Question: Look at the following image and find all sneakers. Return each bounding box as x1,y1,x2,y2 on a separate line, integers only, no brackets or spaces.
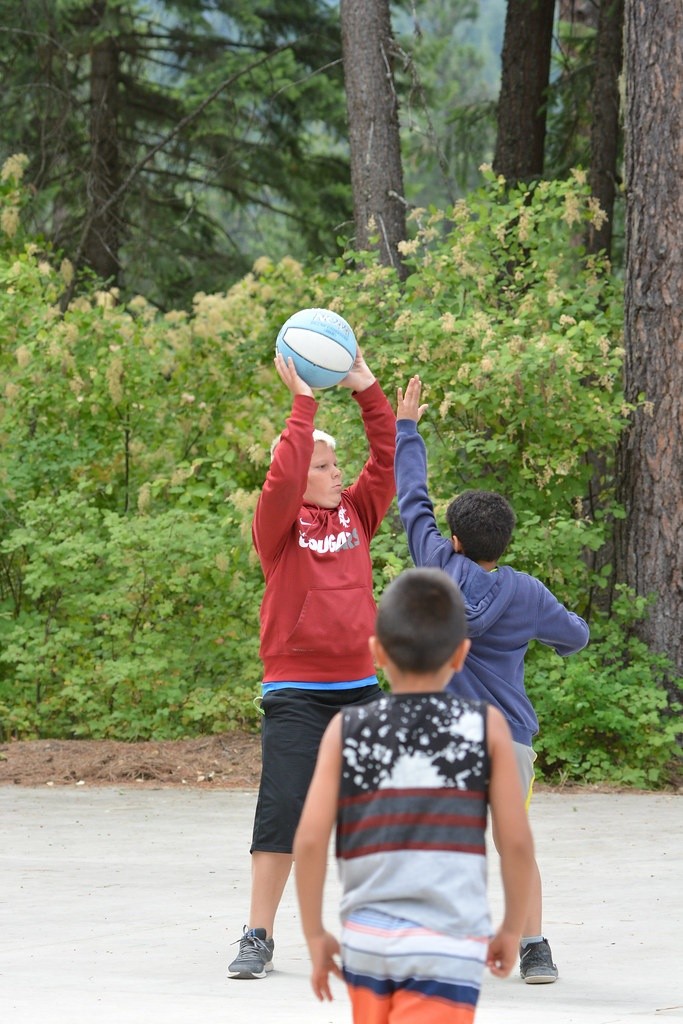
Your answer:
520,937,558,983
227,925,275,978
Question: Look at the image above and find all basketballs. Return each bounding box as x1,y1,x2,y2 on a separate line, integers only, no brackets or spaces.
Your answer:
274,306,359,393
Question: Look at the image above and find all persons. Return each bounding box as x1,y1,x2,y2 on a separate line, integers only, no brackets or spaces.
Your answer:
394,374,590,984
293,566,534,1024
226,343,397,980
